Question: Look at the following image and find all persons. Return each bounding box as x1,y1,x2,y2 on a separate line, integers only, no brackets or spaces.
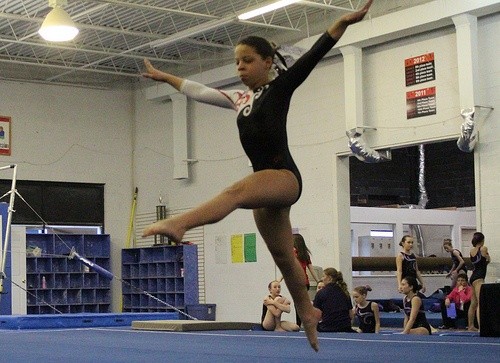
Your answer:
352,285,380,333
467,232,490,331
443,239,467,287
400,276,431,335
310,280,326,305
444,274,473,329
279,234,320,327
261,280,300,333
396,235,426,294
142,0,372,352
313,268,353,333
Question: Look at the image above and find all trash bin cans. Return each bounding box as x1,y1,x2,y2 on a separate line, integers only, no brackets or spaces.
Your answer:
185,303,216,320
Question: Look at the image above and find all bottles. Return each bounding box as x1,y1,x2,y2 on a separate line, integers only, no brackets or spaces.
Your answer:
180,266,184,277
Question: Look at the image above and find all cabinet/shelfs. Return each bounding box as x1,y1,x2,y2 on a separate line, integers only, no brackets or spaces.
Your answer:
122,245,198,312
26,233,114,312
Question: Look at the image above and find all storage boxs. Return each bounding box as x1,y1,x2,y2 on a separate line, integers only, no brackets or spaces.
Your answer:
185,304,216,321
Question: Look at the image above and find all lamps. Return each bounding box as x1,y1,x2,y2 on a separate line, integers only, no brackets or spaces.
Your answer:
38,0,80,42
148,13,237,49
235,0,299,21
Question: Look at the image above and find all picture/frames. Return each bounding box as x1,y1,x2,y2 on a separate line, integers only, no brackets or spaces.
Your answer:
0,116,12,156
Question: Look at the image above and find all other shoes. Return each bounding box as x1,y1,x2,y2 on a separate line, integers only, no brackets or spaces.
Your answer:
439,325,449,329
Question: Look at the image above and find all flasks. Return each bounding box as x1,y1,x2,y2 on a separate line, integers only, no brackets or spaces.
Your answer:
42,276,47,288
